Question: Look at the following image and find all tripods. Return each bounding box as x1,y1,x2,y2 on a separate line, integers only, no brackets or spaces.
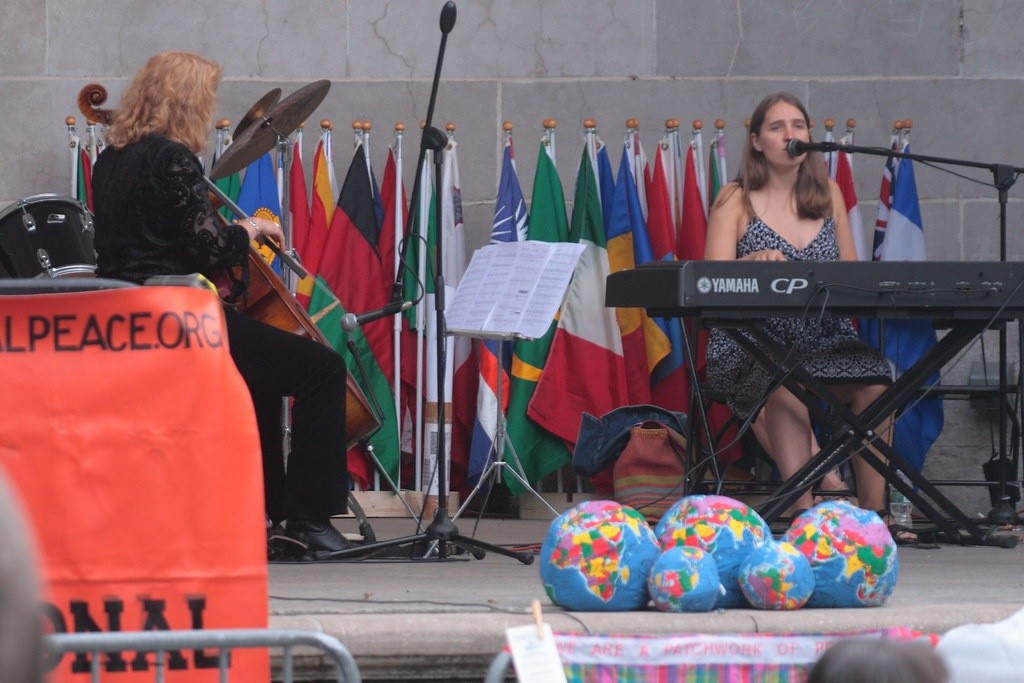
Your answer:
312,0,591,564
822,143,1024,543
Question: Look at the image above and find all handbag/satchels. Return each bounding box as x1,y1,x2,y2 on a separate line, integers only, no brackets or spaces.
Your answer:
614,420,715,522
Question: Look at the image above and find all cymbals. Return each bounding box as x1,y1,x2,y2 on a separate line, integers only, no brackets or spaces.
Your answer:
209,77,333,181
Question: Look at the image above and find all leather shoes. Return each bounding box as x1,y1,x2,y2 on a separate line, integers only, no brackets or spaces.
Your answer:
285,518,381,562
266,522,285,557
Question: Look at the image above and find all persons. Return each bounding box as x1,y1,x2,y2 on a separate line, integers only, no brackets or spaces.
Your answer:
808,637,949,683
704,92,921,547
90,53,369,559
0,465,44,683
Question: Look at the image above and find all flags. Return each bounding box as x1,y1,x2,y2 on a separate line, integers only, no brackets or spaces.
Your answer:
67,115,947,499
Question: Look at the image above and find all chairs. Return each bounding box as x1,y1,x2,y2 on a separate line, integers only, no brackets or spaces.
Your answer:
683,317,890,498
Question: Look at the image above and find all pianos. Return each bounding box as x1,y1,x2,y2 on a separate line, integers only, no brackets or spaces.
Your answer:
602,258,1024,321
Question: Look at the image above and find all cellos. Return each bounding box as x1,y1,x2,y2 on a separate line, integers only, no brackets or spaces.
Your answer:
76,82,441,555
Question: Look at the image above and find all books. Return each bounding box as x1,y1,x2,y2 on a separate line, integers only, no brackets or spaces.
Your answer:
446,240,585,337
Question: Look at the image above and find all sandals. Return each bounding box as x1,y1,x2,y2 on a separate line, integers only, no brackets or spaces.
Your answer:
877,509,919,544
789,509,808,528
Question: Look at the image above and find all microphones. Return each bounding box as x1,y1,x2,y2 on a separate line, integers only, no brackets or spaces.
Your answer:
340,299,420,333
786,138,838,156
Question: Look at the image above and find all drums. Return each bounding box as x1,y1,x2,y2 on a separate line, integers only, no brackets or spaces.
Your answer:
0,191,98,280
